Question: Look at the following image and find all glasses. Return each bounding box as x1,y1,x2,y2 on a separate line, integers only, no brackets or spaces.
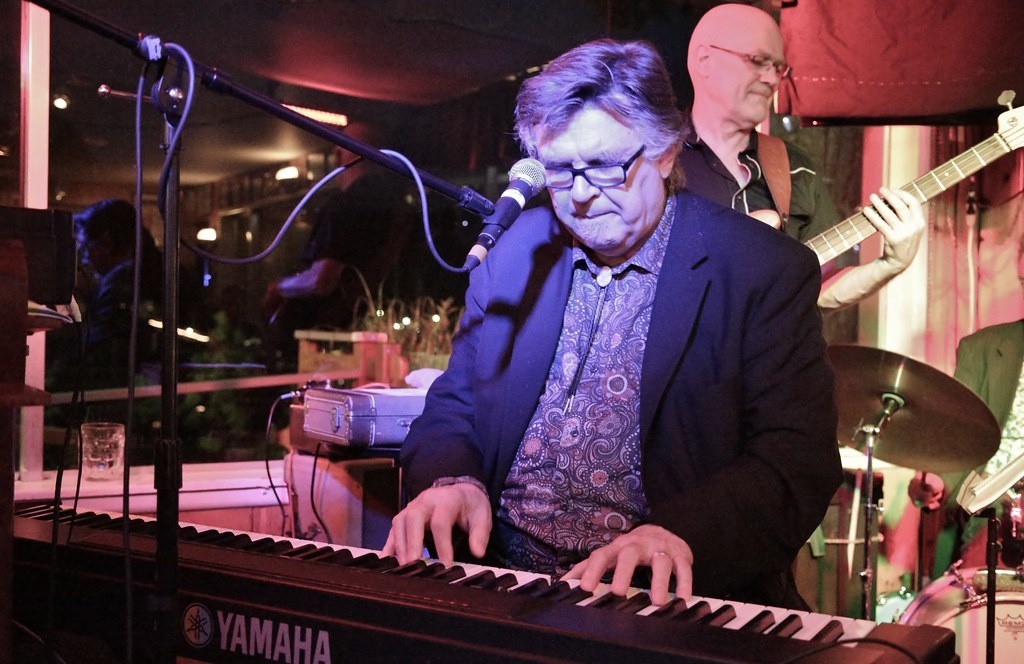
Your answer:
710,44,793,79
543,144,646,189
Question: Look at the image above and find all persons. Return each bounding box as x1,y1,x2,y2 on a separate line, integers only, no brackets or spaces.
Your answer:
265,123,395,372
73,199,164,393
909,233,1024,569
382,38,844,605
675,4,926,315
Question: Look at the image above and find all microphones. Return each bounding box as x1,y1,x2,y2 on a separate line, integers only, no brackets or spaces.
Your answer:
465,158,548,272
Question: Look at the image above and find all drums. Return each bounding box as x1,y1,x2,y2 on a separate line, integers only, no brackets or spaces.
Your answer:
897,564,1024,663
792,469,883,619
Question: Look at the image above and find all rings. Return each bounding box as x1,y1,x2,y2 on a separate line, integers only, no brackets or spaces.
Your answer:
651,549,667,567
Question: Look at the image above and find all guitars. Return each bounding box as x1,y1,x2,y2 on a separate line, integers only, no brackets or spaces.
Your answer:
802,87,1024,266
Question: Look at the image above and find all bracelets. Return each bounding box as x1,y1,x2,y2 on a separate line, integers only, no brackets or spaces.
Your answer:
431,474,490,498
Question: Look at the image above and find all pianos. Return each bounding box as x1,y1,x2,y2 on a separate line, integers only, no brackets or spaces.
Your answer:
10,499,957,663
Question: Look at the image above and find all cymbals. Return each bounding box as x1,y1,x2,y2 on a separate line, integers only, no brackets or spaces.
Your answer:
828,344,1001,471
